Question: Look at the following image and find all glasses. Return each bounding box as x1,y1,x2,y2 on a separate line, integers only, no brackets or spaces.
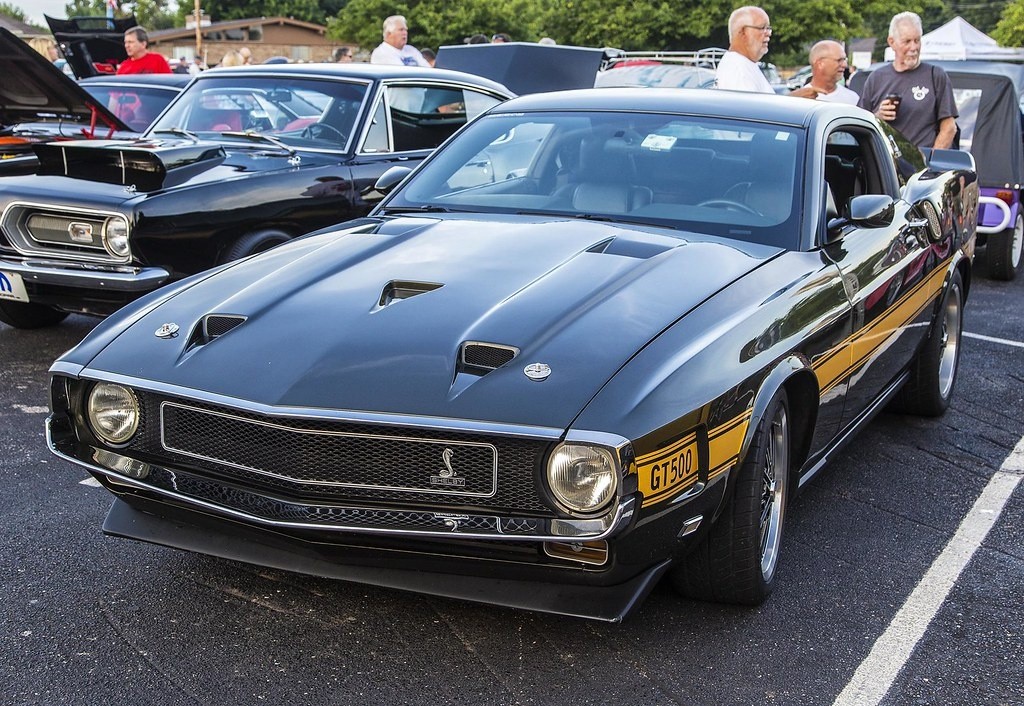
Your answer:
739,24,773,34
820,55,849,65
344,53,353,59
492,35,507,42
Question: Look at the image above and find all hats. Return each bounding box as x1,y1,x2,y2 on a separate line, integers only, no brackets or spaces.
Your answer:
463,34,490,44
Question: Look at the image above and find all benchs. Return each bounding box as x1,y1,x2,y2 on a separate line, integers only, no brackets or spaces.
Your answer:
632,145,854,222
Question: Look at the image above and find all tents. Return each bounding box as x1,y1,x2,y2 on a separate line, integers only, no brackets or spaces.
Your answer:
884,16,997,62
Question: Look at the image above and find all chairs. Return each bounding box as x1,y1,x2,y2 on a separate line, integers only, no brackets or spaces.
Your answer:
719,130,797,222
551,135,653,215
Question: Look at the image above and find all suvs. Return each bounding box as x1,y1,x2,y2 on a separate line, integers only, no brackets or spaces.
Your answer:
592,46,789,102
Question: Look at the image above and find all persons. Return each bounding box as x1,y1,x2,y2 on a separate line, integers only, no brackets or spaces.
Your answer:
860,12,959,150
846,66,858,86
803,40,860,145
54,25,251,129
714,6,818,141
335,15,556,115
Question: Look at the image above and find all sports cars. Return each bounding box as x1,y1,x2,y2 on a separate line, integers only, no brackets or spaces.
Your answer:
0,13,608,329
44,86,981,623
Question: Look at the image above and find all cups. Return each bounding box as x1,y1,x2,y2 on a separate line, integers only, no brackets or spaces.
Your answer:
886,95,902,122
